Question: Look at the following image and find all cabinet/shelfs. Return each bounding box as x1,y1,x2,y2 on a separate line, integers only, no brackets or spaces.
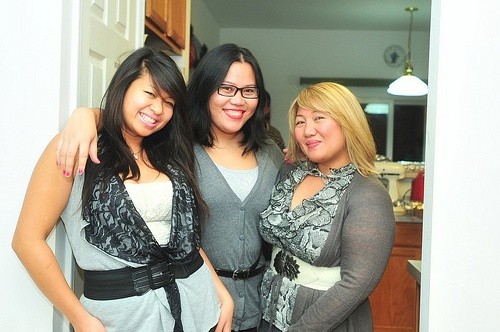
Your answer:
367,223,422,332
145,0,192,83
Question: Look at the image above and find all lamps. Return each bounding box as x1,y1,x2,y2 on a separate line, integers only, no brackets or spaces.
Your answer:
387,6,429,97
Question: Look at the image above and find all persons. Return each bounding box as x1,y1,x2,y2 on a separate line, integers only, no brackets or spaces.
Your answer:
55,43,308,332
259,81,396,332
11,47,235,332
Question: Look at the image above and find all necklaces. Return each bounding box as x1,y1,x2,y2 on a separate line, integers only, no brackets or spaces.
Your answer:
130,149,141,161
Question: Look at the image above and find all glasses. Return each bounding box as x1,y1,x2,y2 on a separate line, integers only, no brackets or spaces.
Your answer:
218,84,259,99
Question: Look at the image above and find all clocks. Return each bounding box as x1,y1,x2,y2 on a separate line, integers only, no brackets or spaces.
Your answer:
383,45,407,68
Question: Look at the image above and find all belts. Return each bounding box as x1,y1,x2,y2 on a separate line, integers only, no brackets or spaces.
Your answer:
214,264,265,280
